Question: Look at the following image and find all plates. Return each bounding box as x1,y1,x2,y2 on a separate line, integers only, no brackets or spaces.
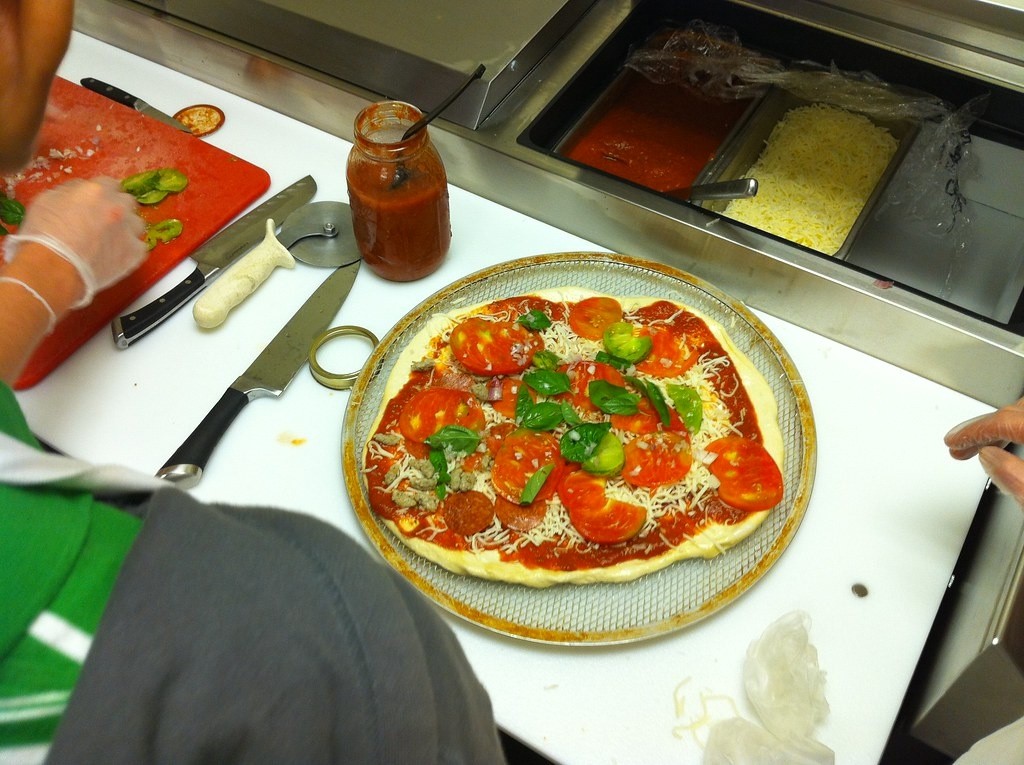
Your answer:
341,252,816,648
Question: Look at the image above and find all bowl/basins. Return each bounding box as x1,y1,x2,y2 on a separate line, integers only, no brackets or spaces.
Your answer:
695,62,917,259
550,28,773,202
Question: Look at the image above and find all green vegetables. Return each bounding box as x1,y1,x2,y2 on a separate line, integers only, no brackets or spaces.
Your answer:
0,191,26,236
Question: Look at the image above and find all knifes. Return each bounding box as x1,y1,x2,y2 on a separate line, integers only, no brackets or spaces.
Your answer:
154,260,360,489
112,176,317,348
80,77,194,134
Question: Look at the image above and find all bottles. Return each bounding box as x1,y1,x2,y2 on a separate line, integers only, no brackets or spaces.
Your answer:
347,101,451,282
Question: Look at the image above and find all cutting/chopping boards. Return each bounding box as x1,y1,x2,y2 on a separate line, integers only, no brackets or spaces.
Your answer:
0,75,268,390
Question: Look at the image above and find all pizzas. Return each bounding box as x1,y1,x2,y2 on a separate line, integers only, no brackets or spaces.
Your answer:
360,284,784,589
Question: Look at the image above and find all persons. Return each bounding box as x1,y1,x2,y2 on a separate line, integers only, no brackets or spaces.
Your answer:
945,395,1024,513
0,1,508,765
